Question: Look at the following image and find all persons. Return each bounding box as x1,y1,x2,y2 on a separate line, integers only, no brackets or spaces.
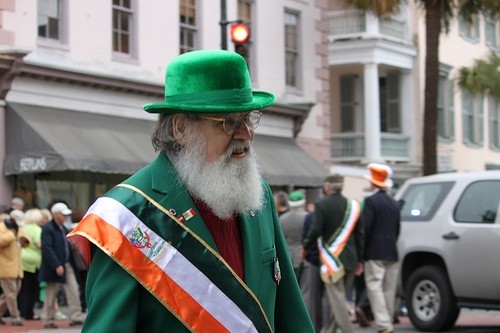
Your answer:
0,209,31,326
300,173,364,332
0,198,89,319
277,191,309,333
273,190,289,216
40,203,87,328
305,199,316,214
359,163,402,333
18,209,43,320
354,275,374,328
82,50,315,333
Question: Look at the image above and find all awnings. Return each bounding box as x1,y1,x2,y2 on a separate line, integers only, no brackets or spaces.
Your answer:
4,101,335,195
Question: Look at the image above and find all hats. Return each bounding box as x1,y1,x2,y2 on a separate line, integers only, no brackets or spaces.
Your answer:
51,202,72,215
363,164,393,188
324,173,345,182
287,191,306,206
142,49,275,112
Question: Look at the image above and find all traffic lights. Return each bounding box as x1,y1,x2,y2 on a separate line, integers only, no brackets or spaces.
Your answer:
230,23,249,42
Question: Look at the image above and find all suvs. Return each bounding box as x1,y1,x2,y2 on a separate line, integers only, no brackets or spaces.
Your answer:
393,170,500,332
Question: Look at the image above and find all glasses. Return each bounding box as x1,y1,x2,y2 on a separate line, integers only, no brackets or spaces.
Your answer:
197,110,264,134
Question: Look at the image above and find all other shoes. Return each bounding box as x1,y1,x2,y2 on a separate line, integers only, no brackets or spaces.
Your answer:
69,320,83,326
0,316,7,325
11,320,23,326
378,326,394,333
54,311,65,318
44,323,58,328
356,307,371,327
